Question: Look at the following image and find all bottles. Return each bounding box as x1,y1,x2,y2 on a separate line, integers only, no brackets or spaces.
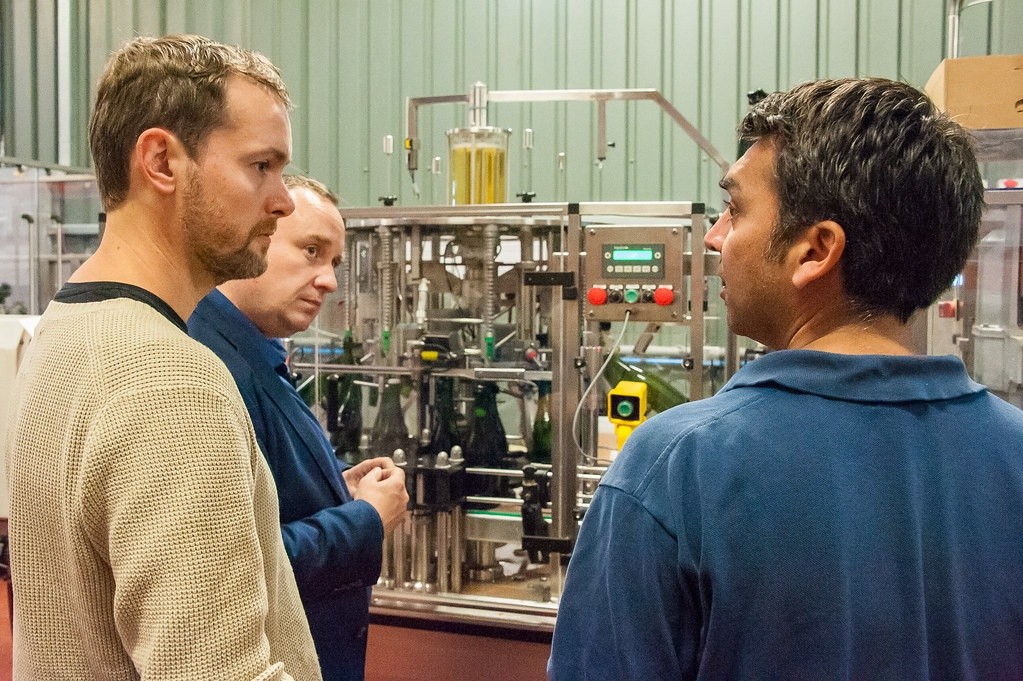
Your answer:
530,382,552,464
421,377,462,505
370,377,409,462
604,356,691,412
328,373,362,466
463,382,507,510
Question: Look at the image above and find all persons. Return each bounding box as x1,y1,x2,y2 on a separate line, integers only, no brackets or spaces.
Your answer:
8,28,329,681
546,73,1023,681
188,172,410,681
